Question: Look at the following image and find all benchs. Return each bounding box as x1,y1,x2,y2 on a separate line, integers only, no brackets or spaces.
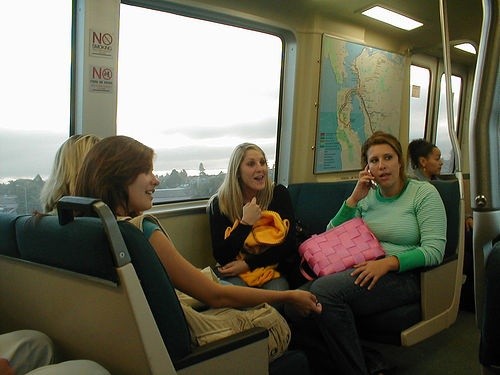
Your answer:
287,171,465,347
0,196,270,375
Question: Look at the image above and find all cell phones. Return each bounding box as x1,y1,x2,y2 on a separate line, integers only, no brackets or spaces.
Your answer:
368,169,378,189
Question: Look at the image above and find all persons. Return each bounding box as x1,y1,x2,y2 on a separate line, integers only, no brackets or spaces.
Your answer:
45,136,321,375
296,130,447,375
0,329,111,375
405,139,443,181
209,143,301,314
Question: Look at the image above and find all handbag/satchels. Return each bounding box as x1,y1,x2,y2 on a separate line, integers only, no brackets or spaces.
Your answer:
298,215,386,281
180,302,293,364
